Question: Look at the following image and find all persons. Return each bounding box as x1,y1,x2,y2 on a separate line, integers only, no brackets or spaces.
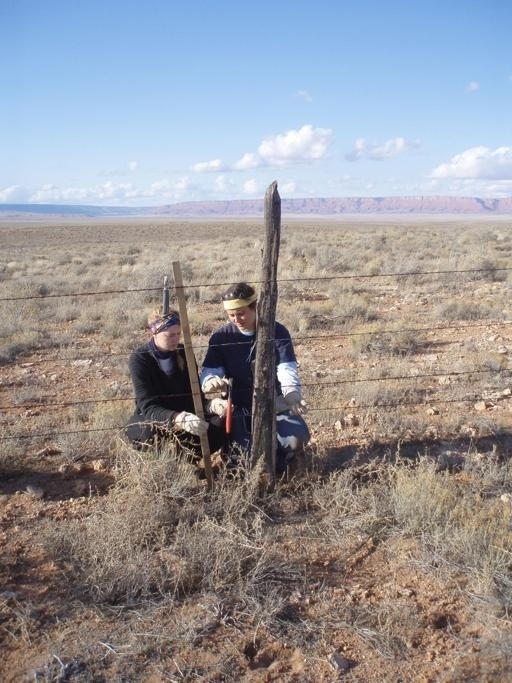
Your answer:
198,282,311,478
126,304,227,462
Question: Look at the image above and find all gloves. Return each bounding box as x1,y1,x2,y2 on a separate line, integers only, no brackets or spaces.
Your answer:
284,390,308,417
174,410,210,436
206,399,235,418
200,374,229,400
